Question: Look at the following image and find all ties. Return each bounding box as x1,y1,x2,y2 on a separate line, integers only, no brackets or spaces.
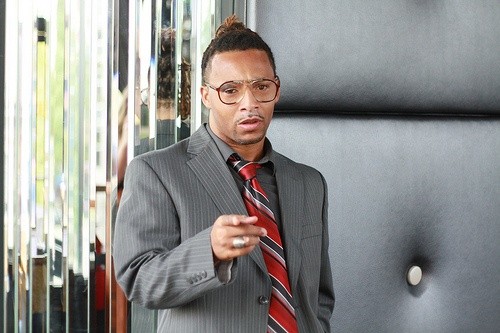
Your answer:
228,155,299,333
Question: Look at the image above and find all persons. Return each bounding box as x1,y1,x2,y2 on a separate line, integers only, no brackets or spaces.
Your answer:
112,15,336,333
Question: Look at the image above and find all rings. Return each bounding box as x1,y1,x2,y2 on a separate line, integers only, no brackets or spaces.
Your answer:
233,236,246,249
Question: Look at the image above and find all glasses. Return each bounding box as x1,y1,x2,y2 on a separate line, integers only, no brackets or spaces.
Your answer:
205,75,280,106
